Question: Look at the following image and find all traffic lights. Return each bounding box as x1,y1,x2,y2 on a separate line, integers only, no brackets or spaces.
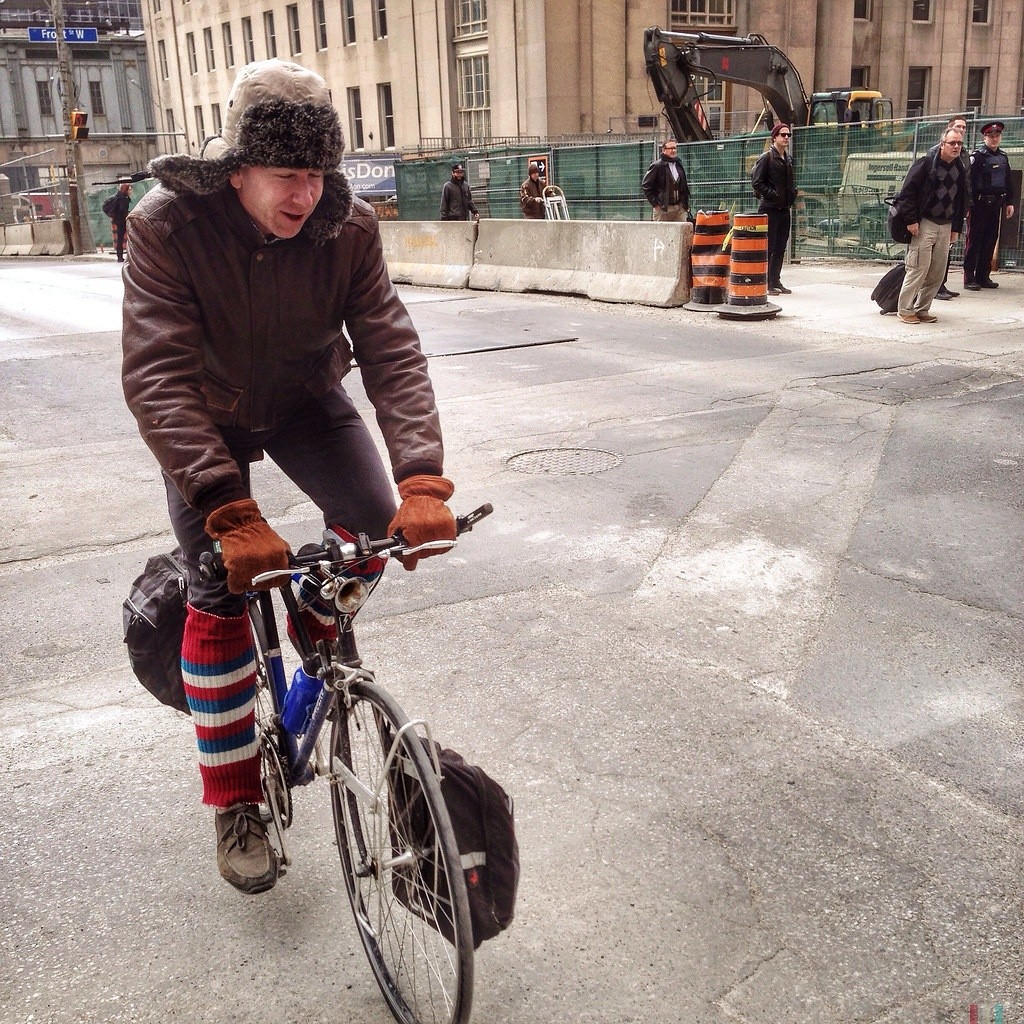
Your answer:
74,126,89,139
72,112,88,126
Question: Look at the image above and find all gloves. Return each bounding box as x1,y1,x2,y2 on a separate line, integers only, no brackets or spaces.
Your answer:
205,499,292,594
550,192,556,197
535,197,544,203
387,475,457,571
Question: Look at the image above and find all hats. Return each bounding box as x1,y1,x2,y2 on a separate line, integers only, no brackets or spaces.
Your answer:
147,57,353,245
529,164,540,174
981,122,1004,135
772,123,791,137
452,164,467,171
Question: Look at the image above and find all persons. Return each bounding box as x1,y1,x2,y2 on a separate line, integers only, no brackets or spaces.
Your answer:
110,182,133,262
120,57,460,896
641,139,692,221
751,123,798,296
926,114,971,300
520,163,556,219
439,164,480,221
896,127,967,325
963,119,1014,291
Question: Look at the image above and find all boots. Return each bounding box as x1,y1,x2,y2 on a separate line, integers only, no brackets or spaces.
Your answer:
117,248,125,262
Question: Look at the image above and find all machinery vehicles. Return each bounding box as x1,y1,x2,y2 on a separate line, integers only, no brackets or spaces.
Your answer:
640,24,913,211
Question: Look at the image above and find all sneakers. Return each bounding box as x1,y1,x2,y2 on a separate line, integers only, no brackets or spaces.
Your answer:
964,280,999,290
897,312,938,323
933,290,960,300
287,622,355,722
769,284,792,296
215,804,278,895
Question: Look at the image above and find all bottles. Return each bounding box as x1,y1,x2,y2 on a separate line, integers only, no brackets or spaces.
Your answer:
281,652,330,732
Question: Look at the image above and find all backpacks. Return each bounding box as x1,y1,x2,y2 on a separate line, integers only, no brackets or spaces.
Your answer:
102,196,120,218
751,150,774,199
123,544,192,716
383,733,521,951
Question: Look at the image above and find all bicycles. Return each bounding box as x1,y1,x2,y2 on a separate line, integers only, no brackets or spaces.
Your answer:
215,422,495,1023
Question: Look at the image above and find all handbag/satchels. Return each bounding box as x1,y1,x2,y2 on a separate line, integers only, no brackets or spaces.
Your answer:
687,212,696,230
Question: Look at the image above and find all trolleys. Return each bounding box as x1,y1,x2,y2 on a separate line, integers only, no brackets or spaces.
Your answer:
538,186,570,221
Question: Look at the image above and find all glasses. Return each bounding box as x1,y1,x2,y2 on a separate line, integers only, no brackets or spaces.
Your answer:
945,140,964,146
664,147,677,150
779,133,792,137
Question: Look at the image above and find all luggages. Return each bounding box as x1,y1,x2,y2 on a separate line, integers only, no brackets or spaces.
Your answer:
871,263,907,315
